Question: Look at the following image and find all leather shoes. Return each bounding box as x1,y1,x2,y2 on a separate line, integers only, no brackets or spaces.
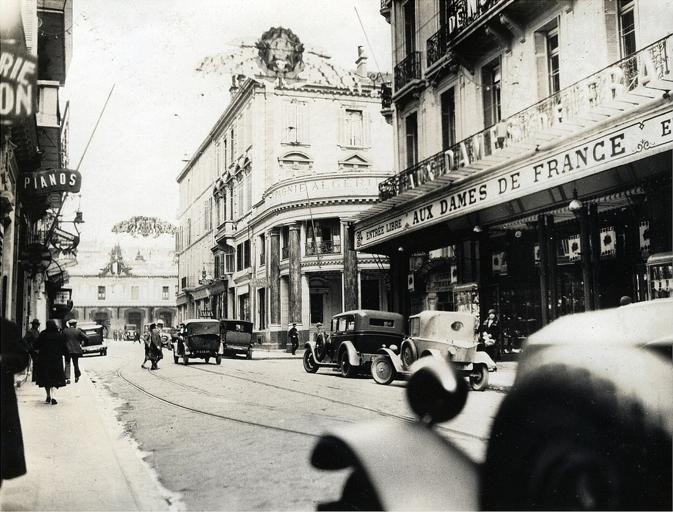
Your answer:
67,378,79,384
46,397,50,403
52,399,57,404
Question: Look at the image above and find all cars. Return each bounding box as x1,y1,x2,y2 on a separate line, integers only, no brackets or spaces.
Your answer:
170,318,223,365
222,320,255,359
303,310,404,375
76,321,107,356
123,324,173,345
370,309,497,389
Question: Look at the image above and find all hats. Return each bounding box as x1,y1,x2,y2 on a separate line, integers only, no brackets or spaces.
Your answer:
68,319,77,323
156,323,164,327
30,319,40,324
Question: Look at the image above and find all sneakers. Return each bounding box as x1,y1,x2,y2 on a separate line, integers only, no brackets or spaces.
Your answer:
141,365,160,369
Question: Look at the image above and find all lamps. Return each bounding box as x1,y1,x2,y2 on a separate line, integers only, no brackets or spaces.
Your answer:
566,199,616,214
471,223,526,236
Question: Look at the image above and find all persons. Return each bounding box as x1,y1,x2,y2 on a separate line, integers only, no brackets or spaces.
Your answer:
103,327,108,339
23,318,89,405
113,321,179,370
288,323,300,355
0,315,29,492
477,309,502,372
312,322,323,341
